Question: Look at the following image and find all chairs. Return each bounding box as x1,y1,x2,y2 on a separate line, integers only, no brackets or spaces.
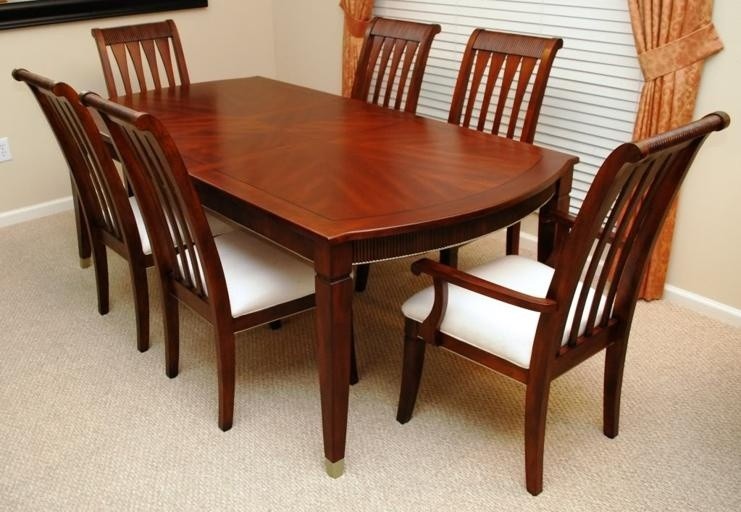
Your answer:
435,26,566,268
89,18,193,197
394,112,730,502
74,86,359,434
10,64,236,357
350,13,443,291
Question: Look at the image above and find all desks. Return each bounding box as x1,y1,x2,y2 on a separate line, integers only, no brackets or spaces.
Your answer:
89,71,583,479
